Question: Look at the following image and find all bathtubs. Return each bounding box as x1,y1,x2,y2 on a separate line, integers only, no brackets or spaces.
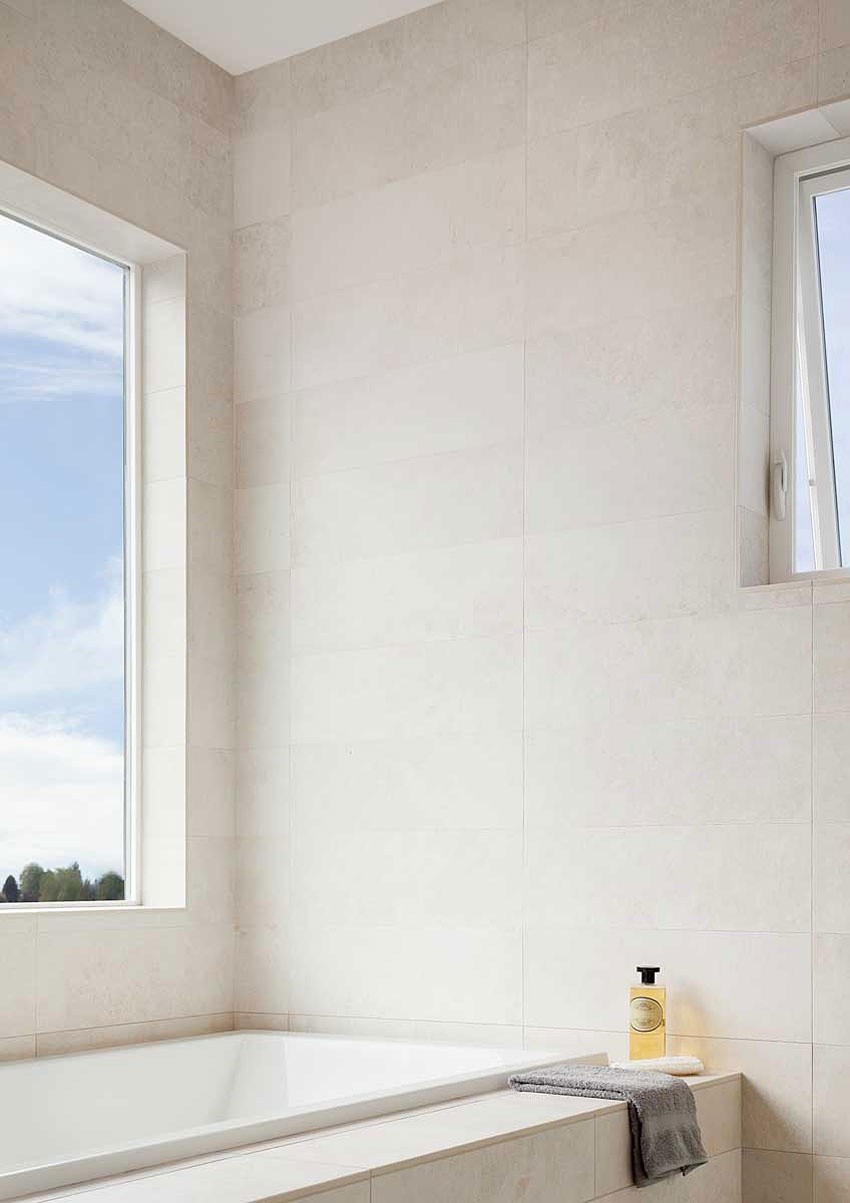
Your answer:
0,1026,587,1200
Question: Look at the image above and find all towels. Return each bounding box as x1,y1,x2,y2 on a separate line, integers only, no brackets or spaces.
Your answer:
609,1053,705,1075
508,1064,711,1190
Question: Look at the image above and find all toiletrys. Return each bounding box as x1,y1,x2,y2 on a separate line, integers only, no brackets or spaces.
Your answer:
627,964,668,1061
